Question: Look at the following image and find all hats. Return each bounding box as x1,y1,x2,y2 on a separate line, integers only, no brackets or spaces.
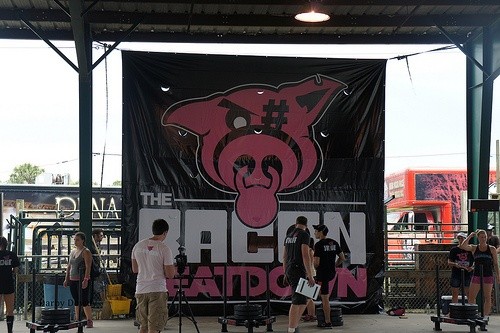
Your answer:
456,232,467,239
313,224,328,236
487,223,495,229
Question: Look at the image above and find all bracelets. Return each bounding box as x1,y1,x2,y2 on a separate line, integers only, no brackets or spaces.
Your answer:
84,278,89,280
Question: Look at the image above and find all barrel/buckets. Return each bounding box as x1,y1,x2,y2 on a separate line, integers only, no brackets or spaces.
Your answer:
43,283,75,320
35,306,45,322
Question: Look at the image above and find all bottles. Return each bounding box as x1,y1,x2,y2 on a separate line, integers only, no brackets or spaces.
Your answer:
426,303,442,315
12,306,27,321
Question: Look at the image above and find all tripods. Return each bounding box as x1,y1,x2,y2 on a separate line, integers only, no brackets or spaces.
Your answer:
168,274,199,333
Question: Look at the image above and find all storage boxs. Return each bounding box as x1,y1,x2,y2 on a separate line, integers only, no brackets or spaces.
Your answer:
441,295,468,316
106,295,132,315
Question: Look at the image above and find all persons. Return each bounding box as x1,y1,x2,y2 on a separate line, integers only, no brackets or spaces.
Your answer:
487,231,500,268
0,236,20,333
312,223,345,328
92,229,105,305
63,232,93,327
132,219,175,333
300,237,317,322
461,230,500,325
448,233,475,303
283,216,315,333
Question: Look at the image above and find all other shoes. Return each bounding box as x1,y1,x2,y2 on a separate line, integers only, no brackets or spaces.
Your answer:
482,315,489,320
86,319,93,328
317,322,333,328
301,314,316,321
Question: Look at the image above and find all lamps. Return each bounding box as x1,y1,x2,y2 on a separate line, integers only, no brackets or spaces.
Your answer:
294,0,330,23
